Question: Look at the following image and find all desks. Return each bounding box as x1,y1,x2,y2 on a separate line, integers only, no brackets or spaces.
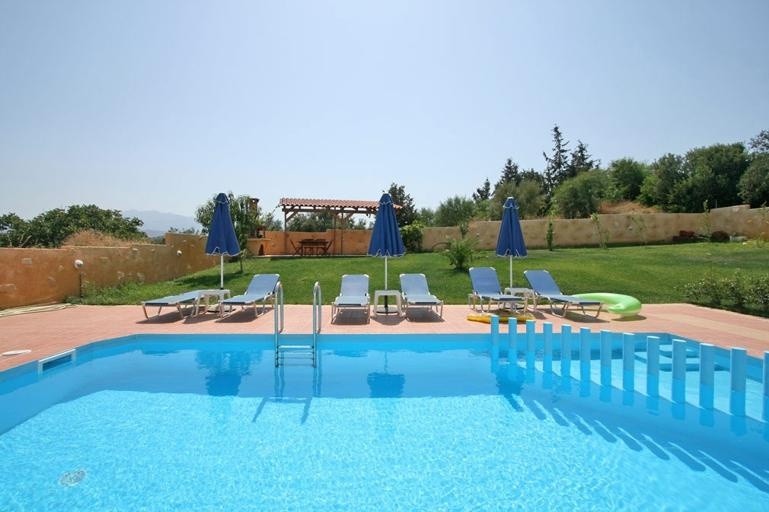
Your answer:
372,288,403,319
298,241,329,256
502,287,539,315
193,289,232,318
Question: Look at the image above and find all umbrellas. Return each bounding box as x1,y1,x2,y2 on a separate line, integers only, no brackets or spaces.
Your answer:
493,194,529,287
365,350,408,444
202,348,243,433
365,192,408,307
202,191,244,289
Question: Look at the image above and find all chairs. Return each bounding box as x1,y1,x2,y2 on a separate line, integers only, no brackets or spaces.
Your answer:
290,239,302,256
217,272,282,322
398,272,444,321
523,268,604,319
466,266,527,316
140,266,231,325
330,273,372,324
321,241,332,256
